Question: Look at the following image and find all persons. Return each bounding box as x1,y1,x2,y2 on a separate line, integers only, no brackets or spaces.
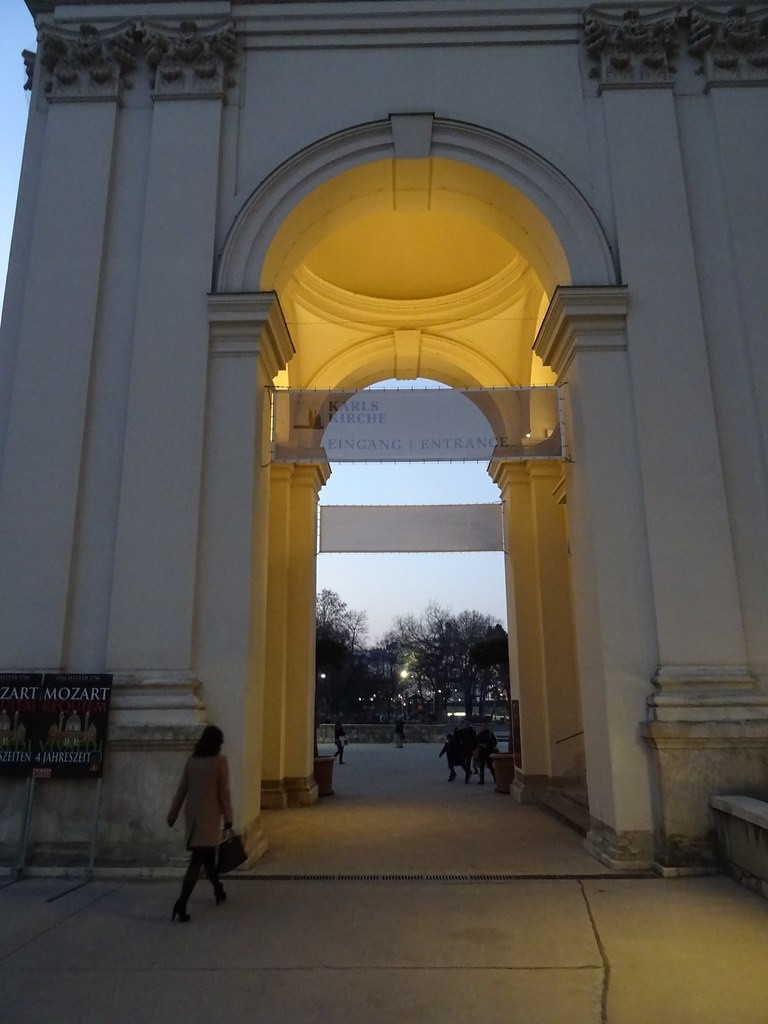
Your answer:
333,717,347,765
438,719,498,784
164,722,236,923
394,715,405,748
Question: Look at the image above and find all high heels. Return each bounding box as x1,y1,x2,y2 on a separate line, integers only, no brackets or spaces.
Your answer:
172,898,190,922
214,882,227,906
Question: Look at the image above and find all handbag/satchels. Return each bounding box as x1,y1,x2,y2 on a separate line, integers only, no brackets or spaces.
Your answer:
493,750,499,753
213,828,247,874
339,734,349,746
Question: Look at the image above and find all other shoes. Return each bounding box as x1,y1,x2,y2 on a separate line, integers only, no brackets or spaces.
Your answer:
448,776,454,782
467,771,472,778
478,781,484,785
339,762,346,764
